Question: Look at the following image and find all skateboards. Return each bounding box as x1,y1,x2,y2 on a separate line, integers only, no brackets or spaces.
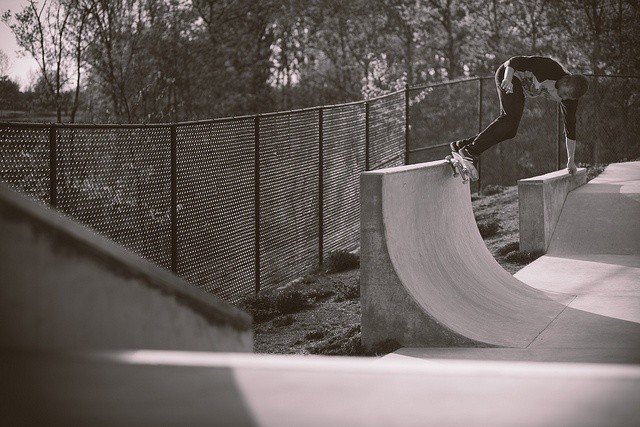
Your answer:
446,152,479,184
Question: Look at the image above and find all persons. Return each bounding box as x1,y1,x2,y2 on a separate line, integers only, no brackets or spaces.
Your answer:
450,56,588,176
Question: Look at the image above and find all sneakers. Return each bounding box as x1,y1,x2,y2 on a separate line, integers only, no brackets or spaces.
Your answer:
449,138,469,153
458,144,480,170
